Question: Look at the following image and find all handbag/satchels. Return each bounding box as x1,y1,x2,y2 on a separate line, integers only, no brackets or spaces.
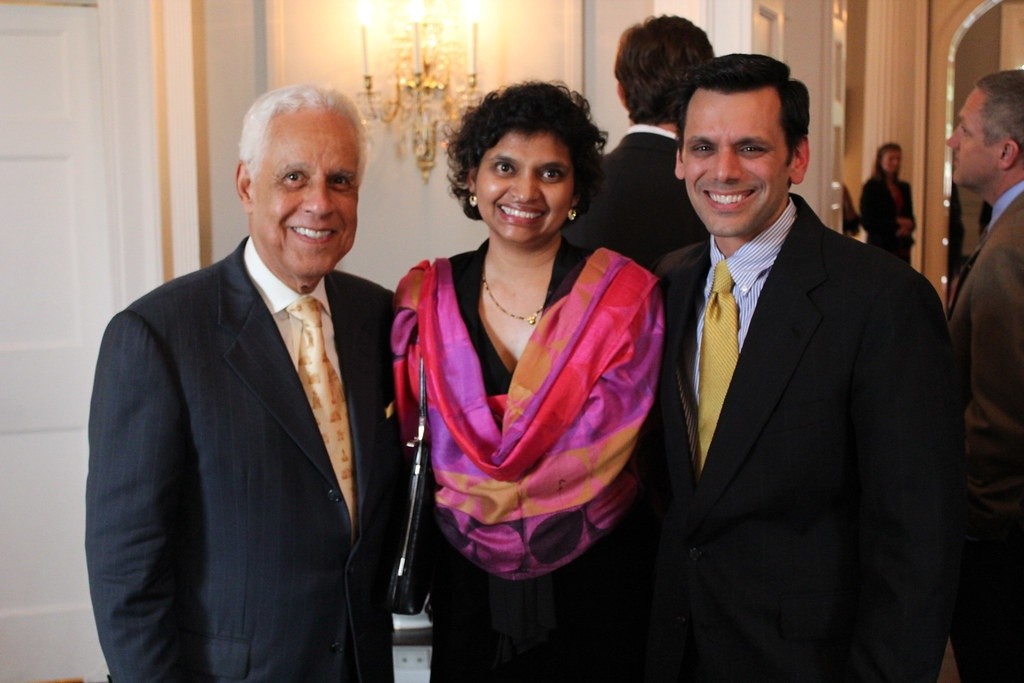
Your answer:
389,441,435,614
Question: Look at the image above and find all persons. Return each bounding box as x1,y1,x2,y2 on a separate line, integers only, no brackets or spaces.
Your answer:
843,185,862,238
390,81,661,683
646,55,961,682
84,85,416,683
562,15,715,268
859,142,915,267
948,67,1024,602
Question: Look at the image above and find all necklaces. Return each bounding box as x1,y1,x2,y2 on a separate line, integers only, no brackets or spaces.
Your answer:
481,272,542,325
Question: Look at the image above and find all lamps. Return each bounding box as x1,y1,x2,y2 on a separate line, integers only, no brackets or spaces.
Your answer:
355,1,490,182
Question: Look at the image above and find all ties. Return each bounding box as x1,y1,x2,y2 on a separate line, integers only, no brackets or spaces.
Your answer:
692,261,737,485
285,293,356,544
